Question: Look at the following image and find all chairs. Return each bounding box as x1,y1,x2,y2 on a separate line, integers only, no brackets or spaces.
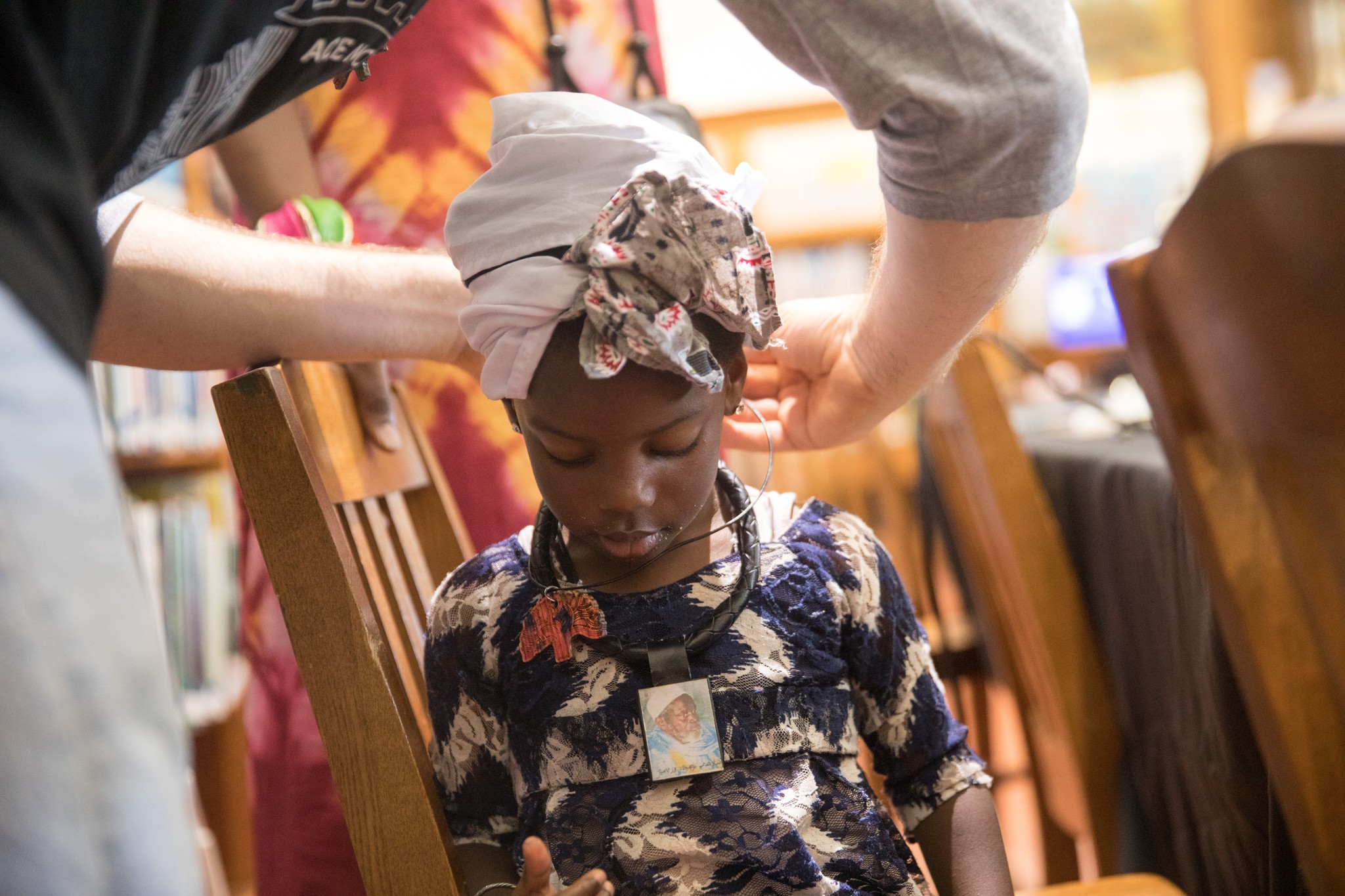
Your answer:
212,360,491,896
916,334,1185,895
1105,95,1345,896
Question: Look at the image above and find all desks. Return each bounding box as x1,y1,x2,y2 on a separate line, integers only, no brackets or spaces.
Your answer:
919,379,1312,896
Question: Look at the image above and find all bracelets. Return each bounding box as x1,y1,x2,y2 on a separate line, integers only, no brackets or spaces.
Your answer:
237,194,358,249
474,883,518,896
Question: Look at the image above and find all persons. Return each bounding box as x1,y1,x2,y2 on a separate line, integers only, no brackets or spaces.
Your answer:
424,93,1014,896
0,0,1090,896
210,0,668,896
647,684,722,780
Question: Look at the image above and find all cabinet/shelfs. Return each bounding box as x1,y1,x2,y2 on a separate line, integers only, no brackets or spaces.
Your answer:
84,144,261,896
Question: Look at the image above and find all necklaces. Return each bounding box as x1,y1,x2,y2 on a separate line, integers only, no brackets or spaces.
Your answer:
538,0,661,103
531,458,760,780
